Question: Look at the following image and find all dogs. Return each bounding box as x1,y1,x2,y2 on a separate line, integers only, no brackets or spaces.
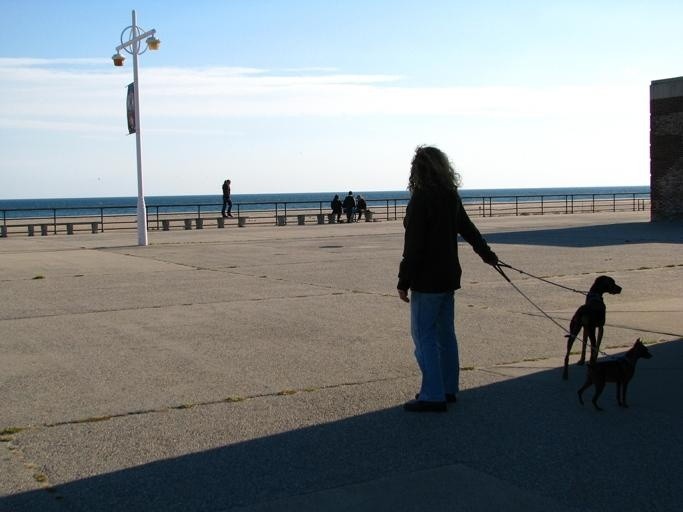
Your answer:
561,275,623,380
575,337,655,411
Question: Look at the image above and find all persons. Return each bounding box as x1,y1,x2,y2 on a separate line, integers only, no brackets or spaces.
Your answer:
398,147,498,413
331,195,343,221
222,179,233,218
343,191,356,223
356,195,366,222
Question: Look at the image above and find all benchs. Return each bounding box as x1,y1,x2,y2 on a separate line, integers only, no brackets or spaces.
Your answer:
161,214,249,231
0,221,102,237
273,210,375,227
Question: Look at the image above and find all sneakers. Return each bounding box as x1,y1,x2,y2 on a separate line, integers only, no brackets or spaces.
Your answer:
404,401,448,413
415,393,455,402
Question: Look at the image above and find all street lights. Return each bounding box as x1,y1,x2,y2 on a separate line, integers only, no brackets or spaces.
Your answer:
112,10,161,245
